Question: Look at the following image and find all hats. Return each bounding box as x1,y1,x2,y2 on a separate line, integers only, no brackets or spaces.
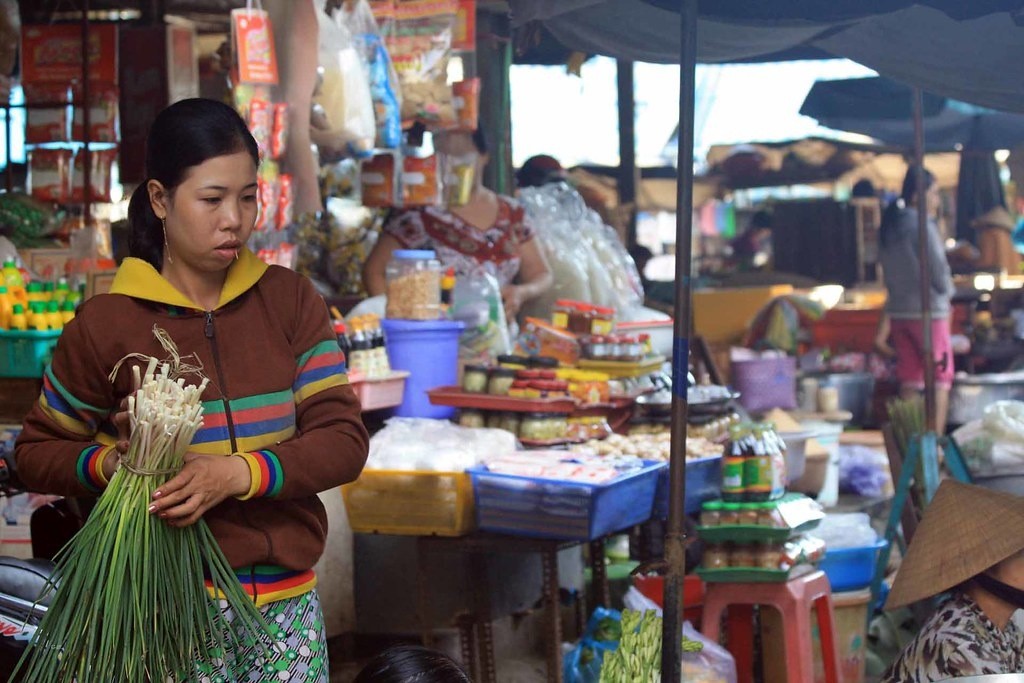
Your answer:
515,156,569,179
970,205,1016,232
882,479,1024,609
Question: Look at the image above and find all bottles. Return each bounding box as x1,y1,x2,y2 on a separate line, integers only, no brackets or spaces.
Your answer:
0,256,75,331
386,248,442,321
718,418,789,502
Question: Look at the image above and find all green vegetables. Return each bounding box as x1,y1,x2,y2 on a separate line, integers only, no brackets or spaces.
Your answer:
598,608,704,683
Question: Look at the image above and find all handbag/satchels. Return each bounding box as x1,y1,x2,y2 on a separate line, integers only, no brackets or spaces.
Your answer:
313,0,402,153
562,587,737,683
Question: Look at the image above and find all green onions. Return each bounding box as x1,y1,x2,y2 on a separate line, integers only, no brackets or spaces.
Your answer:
7,356,286,683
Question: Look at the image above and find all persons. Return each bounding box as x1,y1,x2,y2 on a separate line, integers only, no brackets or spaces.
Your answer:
875,301,897,359
363,114,553,340
875,165,957,464
11,98,369,683
725,210,772,260
353,646,470,683
881,479,1024,683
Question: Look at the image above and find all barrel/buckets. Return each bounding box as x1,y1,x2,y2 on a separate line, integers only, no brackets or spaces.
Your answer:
377,317,465,419
799,422,842,505
633,575,703,626
732,356,796,411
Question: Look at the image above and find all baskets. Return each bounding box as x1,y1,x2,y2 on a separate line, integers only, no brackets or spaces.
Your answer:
732,336,795,414
341,417,516,537
775,430,822,485
463,438,723,543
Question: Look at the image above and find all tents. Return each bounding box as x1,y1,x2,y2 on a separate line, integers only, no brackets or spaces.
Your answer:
481,0,1024,683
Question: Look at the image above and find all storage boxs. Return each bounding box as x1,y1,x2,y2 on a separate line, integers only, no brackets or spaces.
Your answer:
340,468,475,536
0,329,63,378
18,249,72,282
654,456,723,520
465,454,668,540
350,370,409,409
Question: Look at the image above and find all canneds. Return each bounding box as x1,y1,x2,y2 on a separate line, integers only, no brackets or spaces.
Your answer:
460,355,570,443
700,491,811,570
798,376,838,413
551,298,618,336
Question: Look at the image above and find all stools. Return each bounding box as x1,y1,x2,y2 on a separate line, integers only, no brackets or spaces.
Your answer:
703,571,842,683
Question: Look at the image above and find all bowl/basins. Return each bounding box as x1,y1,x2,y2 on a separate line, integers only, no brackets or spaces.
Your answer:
808,540,888,588
